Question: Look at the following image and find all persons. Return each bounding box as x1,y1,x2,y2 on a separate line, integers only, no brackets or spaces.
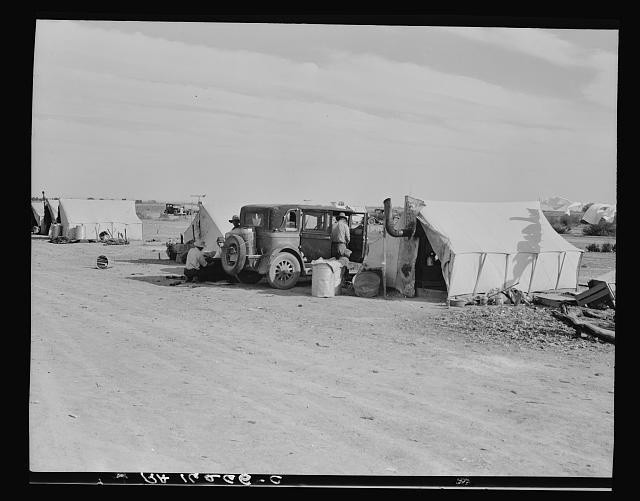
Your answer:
184,241,208,283
331,212,350,260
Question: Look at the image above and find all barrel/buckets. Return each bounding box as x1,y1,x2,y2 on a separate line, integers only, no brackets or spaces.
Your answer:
75,225,86,240
310,262,335,299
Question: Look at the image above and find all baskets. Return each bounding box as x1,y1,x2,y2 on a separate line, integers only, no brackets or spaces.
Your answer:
352,270,380,296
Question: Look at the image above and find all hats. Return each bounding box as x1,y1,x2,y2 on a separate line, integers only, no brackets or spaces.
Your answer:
193,240,205,247
229,215,239,222
336,213,347,221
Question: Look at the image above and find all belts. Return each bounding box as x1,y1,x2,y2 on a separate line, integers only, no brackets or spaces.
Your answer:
333,242,344,243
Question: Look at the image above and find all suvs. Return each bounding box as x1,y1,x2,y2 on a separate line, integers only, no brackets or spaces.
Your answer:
165,204,180,214
216,204,368,290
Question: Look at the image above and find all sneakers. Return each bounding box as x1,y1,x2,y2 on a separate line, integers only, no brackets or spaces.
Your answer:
186,278,192,282
193,277,200,282
